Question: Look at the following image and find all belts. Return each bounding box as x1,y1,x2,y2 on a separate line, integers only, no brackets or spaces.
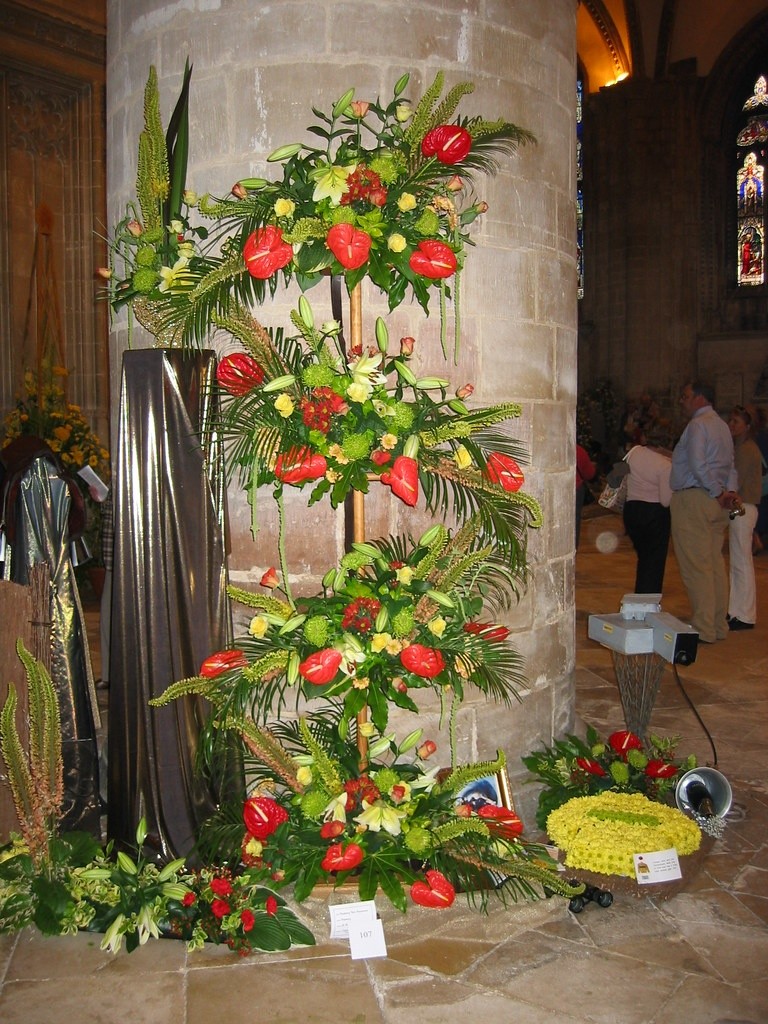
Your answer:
675,486,700,491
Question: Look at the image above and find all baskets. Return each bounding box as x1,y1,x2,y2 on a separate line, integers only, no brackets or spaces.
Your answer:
558,798,717,904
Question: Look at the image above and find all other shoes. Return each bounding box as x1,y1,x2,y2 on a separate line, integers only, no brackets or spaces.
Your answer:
726,613,754,630
698,633,728,644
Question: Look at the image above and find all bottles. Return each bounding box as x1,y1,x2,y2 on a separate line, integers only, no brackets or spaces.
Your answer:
686,781,714,818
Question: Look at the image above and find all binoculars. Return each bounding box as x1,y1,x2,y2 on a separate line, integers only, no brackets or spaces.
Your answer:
543,878,613,914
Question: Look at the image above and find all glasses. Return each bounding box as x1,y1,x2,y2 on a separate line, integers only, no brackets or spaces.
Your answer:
735,404,745,412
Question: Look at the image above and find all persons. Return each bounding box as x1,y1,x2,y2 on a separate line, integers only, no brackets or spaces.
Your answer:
669,379,743,643
726,403,763,631
623,421,674,594
576,444,596,552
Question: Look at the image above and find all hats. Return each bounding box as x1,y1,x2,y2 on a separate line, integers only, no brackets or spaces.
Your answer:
742,400,760,431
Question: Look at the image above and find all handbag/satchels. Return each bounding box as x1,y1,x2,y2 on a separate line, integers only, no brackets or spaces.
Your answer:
597,445,642,515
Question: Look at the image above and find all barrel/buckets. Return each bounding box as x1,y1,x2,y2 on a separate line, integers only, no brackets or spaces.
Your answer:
675,767,733,819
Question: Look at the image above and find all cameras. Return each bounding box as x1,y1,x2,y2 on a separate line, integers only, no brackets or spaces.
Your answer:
729,499,745,520
